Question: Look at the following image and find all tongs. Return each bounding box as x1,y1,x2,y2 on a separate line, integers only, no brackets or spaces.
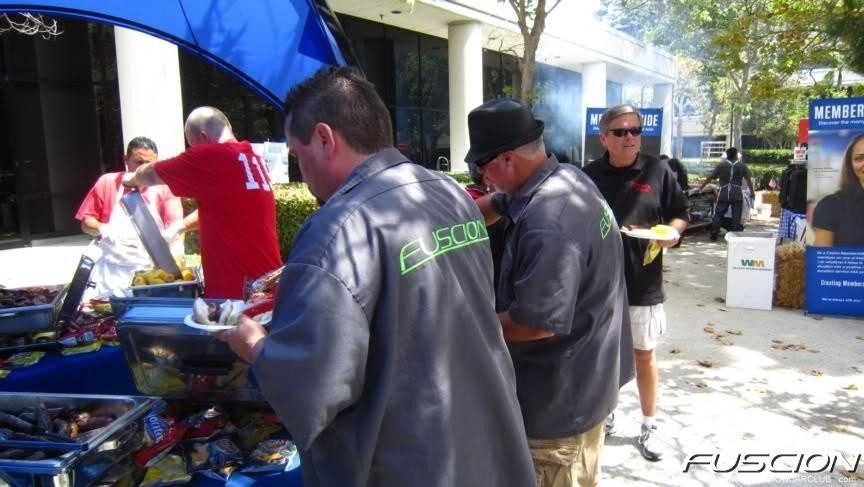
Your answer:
251,264,287,292
0,411,89,452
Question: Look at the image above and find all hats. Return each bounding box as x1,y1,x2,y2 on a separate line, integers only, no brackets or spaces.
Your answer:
465,98,545,165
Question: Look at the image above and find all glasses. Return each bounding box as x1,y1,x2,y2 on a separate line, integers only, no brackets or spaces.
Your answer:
607,125,643,137
474,148,515,168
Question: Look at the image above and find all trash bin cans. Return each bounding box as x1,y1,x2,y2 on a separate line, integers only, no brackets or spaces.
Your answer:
724,231,777,311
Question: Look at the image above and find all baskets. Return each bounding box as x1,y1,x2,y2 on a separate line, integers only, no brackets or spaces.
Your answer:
763,190,780,216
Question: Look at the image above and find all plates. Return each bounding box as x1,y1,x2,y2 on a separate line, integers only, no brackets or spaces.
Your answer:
620,228,680,241
181,305,273,330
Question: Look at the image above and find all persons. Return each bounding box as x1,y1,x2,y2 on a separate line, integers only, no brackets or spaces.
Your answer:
72,136,186,296
213,64,539,487
699,147,754,240
812,132,864,246
465,97,632,487
581,101,694,462
661,155,690,190
121,104,287,299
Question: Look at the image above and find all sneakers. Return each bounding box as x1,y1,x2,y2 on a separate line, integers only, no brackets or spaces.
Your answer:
605,414,617,437
638,423,664,462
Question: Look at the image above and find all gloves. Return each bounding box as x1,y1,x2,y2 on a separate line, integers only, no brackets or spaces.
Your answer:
99,224,120,248
161,224,180,249
